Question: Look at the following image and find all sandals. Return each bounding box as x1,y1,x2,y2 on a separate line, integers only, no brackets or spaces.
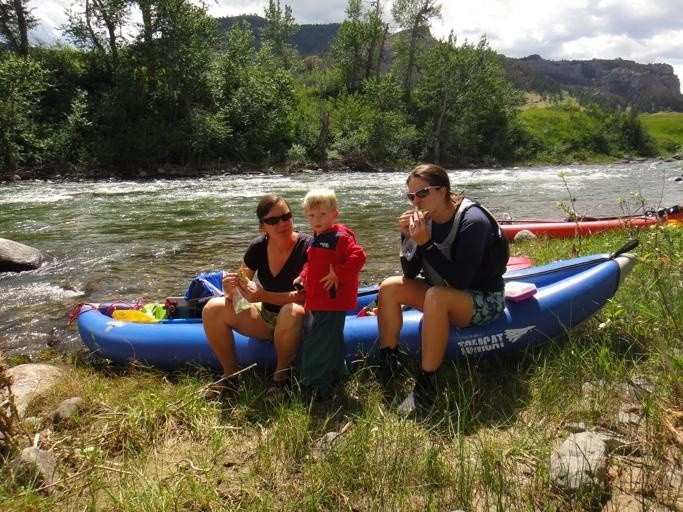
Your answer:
202,376,238,399
265,377,286,408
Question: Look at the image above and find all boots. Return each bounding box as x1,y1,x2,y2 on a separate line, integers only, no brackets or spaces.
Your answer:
379,345,403,386
414,369,437,412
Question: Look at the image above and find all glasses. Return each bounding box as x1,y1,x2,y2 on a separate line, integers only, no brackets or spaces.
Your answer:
407,185,440,201
261,211,292,225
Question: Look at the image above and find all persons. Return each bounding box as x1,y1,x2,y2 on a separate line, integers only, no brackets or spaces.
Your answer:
287,189,367,403
201,195,313,404
376,165,509,417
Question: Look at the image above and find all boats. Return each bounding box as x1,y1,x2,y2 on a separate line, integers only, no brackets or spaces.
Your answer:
496,205,683,244
78,239,641,380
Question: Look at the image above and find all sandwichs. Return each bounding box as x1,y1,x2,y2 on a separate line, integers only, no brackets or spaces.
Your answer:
418,209,431,223
237,267,250,287
